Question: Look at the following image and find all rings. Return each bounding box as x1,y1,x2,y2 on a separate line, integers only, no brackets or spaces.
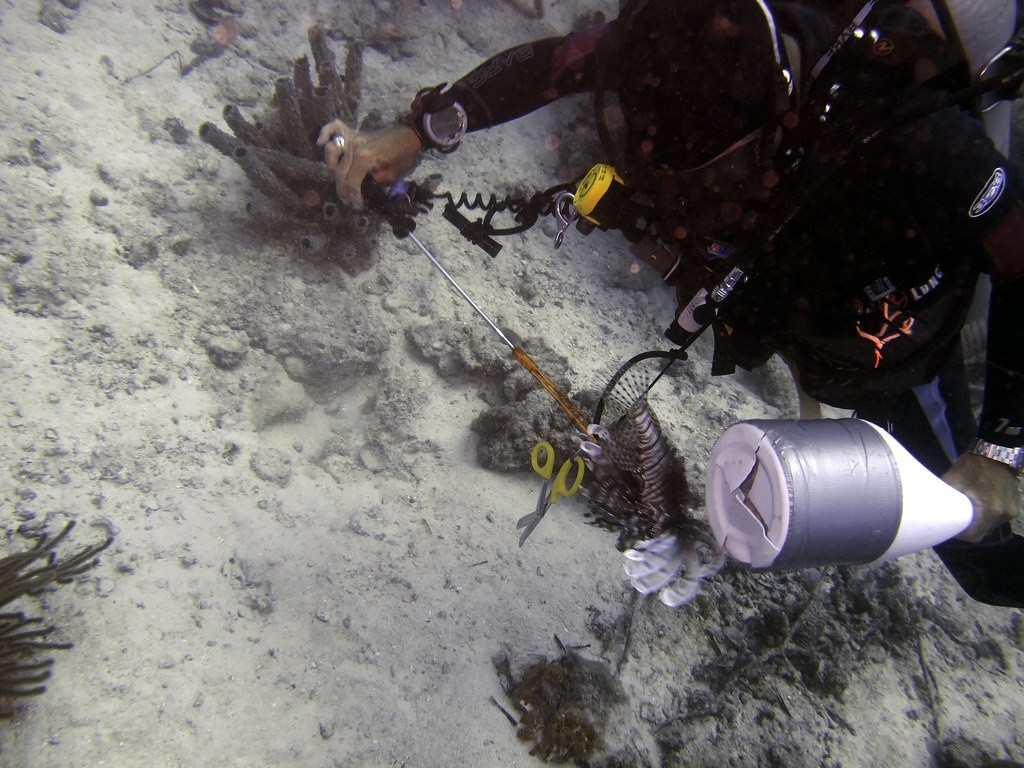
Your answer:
333,136,344,153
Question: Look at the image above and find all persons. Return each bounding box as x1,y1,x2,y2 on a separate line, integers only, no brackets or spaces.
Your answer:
317,0,1024,609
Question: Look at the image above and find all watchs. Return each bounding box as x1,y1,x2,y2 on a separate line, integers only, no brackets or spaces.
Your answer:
422,100,468,153
967,437,1024,472
